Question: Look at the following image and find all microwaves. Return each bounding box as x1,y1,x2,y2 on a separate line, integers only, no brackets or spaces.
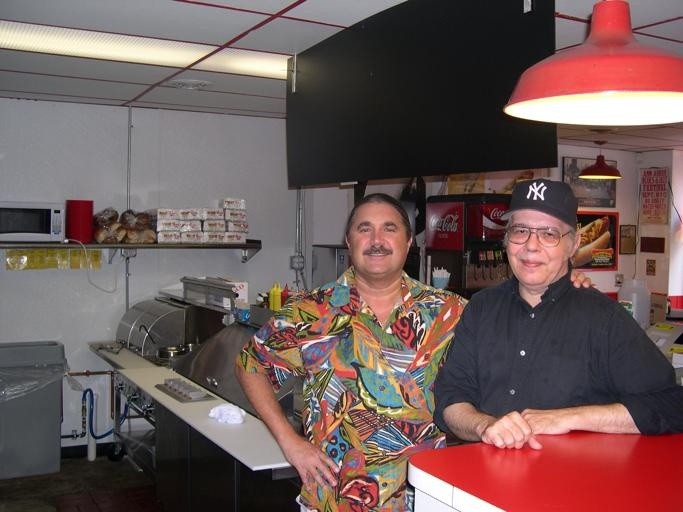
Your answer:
0,200,66,244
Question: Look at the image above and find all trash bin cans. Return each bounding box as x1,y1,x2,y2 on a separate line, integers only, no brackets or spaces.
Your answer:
0,341,65,480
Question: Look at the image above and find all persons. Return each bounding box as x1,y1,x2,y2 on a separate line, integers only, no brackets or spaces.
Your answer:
232,191,602,511
431,177,682,448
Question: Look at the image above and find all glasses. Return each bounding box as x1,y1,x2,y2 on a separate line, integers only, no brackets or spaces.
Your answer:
508,226,572,247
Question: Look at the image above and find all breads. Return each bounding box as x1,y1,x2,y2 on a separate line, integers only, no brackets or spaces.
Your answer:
94,208,156,244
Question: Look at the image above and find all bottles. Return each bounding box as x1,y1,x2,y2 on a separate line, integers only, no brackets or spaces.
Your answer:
262,283,289,312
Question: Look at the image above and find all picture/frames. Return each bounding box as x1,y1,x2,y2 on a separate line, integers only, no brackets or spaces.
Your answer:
562,156,618,209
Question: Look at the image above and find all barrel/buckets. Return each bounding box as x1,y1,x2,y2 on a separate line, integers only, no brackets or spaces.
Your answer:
617,279,650,330
65,199,93,244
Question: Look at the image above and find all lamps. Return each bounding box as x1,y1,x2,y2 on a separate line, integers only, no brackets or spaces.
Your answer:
578,141,624,180
503,0,683,127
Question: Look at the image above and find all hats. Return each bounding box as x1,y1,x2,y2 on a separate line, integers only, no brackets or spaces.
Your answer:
499,179,578,231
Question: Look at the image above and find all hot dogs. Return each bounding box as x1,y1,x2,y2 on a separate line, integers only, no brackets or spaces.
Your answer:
571,217,611,267
